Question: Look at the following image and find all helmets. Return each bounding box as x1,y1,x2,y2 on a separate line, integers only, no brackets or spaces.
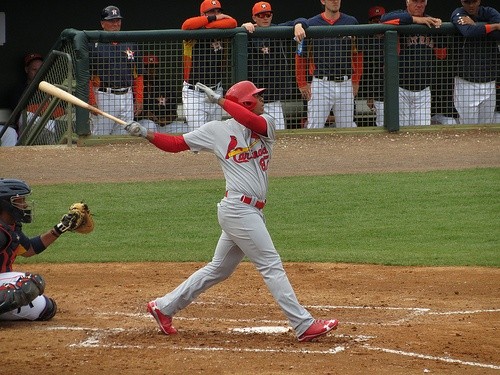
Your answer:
224,80,267,110
0,178,33,224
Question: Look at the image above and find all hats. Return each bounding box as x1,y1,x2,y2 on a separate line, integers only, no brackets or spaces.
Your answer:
367,6,385,19
99,5,123,20
140,55,160,66
208,9,222,13
23,52,44,65
252,1,271,15
200,0,221,13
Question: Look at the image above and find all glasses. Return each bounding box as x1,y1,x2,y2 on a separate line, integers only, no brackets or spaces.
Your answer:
255,13,272,18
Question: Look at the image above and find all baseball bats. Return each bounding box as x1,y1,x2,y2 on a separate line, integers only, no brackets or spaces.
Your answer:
38,80,139,134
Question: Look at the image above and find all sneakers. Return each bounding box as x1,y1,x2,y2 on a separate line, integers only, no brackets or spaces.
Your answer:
298,318,338,342
148,299,177,336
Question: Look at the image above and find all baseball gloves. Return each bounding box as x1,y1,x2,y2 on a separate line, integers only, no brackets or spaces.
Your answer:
54,202,95,235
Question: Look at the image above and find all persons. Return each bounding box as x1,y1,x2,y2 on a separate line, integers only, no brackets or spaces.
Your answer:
10,51,66,146
449,0,500,126
0,125,18,146
123,80,340,344
240,2,310,132
181,0,238,133
361,6,388,127
429,46,500,126
86,6,144,137
379,0,442,126
295,0,364,128
0,178,94,323
135,54,190,136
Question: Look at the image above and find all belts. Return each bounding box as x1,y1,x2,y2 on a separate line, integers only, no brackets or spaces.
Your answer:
314,75,350,81
97,87,129,94
225,191,265,208
188,83,221,92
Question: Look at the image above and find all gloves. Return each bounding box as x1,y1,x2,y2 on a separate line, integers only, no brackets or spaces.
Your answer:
122,120,148,139
54,210,81,234
196,81,220,105
